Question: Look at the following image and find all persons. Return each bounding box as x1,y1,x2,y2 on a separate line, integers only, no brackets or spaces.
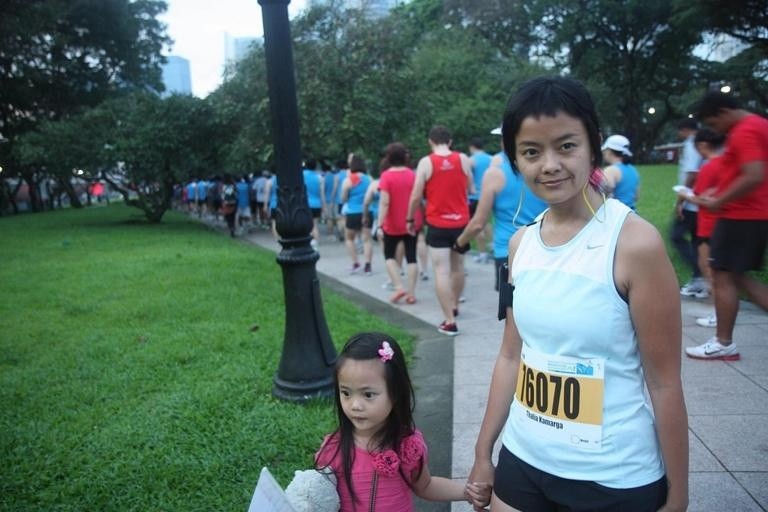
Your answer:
451,121,549,290
598,135,641,213
466,73,688,512
466,138,495,264
407,126,472,336
673,93,768,359
313,332,492,512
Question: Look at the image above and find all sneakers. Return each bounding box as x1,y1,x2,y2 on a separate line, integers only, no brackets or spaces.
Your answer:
364,268,372,275
390,290,406,302
679,283,709,299
407,294,417,304
685,335,740,362
349,263,361,274
438,318,458,337
696,315,719,328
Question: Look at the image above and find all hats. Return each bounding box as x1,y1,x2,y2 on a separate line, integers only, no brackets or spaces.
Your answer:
601,133,631,153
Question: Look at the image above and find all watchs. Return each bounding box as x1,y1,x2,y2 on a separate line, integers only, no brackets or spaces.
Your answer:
453,241,470,254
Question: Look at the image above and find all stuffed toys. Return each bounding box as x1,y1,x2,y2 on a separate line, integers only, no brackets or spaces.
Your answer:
285,466,340,512
